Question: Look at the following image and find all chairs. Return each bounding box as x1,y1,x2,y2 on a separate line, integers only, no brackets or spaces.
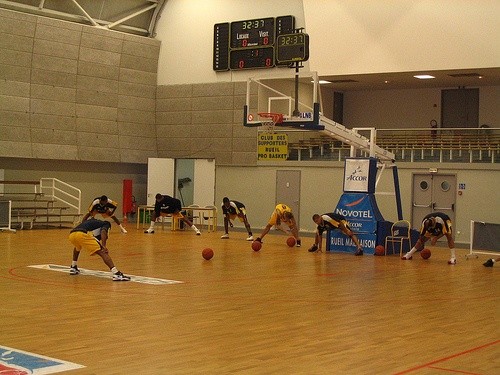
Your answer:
201,206,218,233
162,215,172,231
186,204,202,232
385,220,412,257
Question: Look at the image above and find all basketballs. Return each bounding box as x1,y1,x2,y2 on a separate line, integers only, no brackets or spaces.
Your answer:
287,237,296,247
375,245,384,255
252,241,262,251
202,248,214,260
420,249,431,260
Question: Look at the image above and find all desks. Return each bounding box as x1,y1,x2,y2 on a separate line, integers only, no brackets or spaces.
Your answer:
136,206,216,233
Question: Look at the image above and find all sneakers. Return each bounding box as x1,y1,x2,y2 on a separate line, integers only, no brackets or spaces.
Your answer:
401,252,412,260
252,237,261,243
196,230,200,234
448,258,456,264
246,236,254,240
112,271,130,281
355,248,363,256
70,264,79,274
482,259,494,267
308,244,318,252
144,230,154,233
297,240,301,246
221,234,229,238
122,228,127,233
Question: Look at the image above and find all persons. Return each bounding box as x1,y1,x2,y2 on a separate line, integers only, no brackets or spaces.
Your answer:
82,195,128,234
400,213,456,265
68,207,131,281
482,256,500,267
221,197,254,241
145,194,201,235
255,204,301,247
308,213,363,256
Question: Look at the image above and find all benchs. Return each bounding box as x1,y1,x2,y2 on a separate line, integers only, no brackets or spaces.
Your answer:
288,133,500,164
0,193,84,230
